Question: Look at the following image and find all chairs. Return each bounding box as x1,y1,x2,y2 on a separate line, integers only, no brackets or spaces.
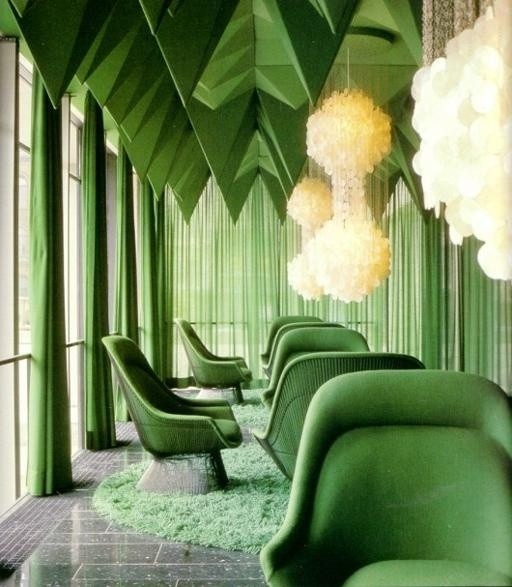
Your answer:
261,316,370,408
259,370,512,587
100,320,255,499
255,352,426,481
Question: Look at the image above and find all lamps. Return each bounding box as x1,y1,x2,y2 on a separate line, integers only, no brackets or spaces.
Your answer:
288,176,332,302
410,0,512,280
305,48,394,304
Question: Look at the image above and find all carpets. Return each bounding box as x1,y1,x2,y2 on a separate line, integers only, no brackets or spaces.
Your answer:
90,384,292,554
